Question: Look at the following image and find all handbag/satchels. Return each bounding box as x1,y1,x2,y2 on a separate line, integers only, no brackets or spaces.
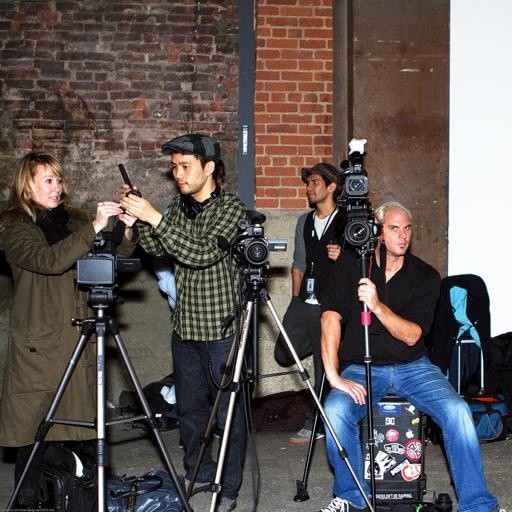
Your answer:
12,441,184,512
429,396,507,444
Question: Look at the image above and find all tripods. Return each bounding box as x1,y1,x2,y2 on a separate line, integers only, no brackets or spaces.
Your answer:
186,267,375,512
5,288,195,512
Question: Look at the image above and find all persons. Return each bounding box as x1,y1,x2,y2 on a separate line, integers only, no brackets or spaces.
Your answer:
318,197,505,511
0,150,139,511
270,162,357,445
118,133,250,512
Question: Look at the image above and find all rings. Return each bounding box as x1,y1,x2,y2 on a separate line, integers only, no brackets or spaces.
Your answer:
353,384,358,390
102,201,106,205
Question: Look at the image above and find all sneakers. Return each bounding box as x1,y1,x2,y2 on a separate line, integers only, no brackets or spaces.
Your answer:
111,473,162,499
183,473,213,495
215,496,237,512
319,496,367,512
289,427,325,444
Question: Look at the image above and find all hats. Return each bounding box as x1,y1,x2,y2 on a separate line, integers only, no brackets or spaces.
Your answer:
301,162,346,185
161,133,221,161
